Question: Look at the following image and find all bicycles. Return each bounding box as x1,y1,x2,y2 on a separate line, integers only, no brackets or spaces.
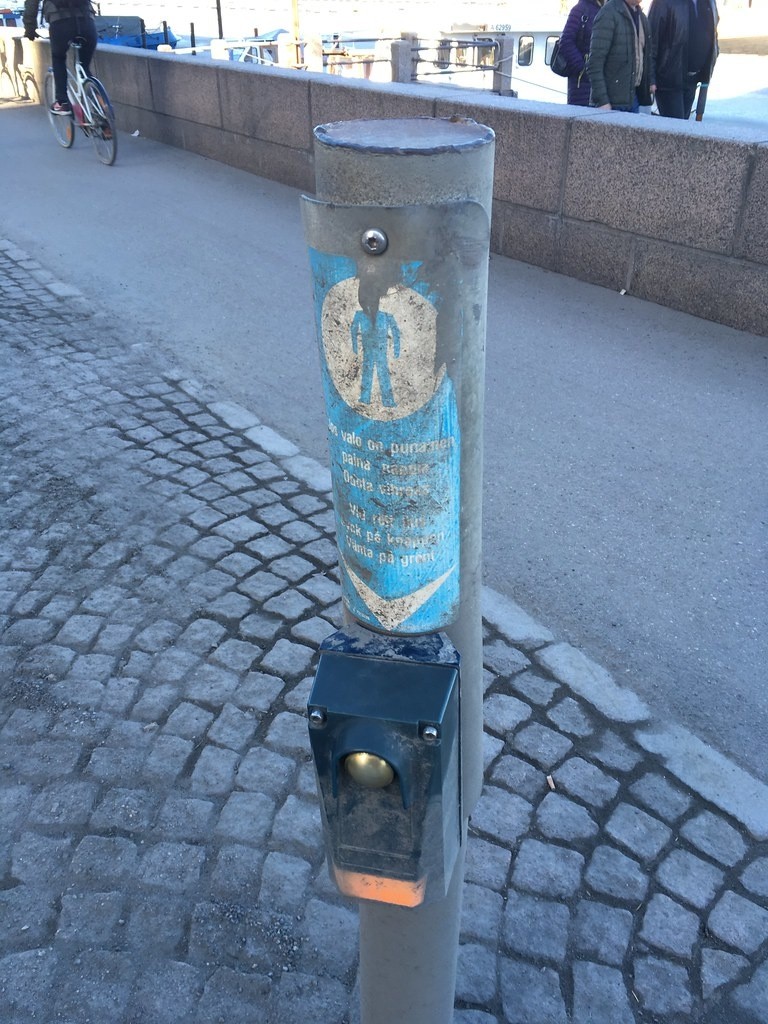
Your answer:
24,31,118,166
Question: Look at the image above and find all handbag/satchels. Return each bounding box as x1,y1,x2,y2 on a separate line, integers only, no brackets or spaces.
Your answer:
551,2,589,76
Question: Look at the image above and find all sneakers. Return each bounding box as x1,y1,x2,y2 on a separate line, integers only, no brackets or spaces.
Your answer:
103,127,112,138
50,100,72,116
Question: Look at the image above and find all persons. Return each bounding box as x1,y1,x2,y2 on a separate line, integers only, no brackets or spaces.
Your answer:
559,0,611,108
22,0,114,140
647,0,720,121
589,0,651,113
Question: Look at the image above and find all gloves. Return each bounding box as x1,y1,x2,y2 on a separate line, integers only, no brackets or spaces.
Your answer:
25,28,35,40
